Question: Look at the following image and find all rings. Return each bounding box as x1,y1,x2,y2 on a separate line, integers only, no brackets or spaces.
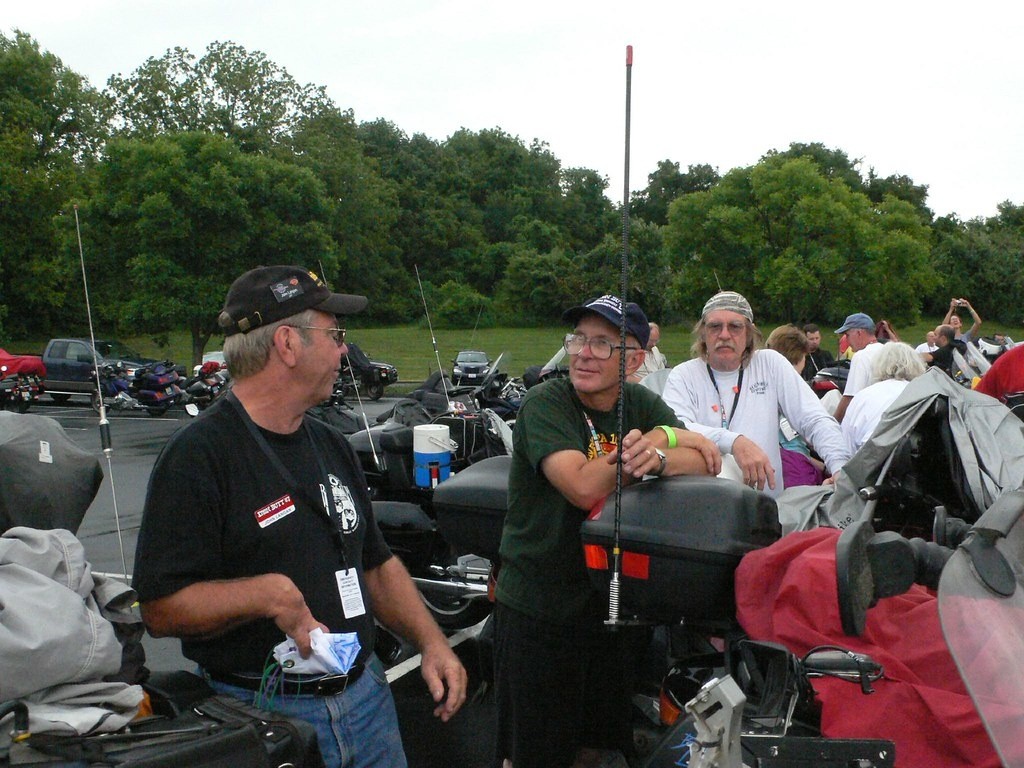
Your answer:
646,450,651,455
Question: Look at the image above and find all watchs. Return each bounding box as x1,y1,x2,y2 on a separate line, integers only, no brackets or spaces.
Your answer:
655,448,667,476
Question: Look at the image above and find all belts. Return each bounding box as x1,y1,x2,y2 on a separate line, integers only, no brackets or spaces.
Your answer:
205,659,365,698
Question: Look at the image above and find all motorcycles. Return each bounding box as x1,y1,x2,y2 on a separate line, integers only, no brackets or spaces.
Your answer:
0,338,1024,768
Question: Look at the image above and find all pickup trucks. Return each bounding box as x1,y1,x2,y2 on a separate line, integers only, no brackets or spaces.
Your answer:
12,336,185,403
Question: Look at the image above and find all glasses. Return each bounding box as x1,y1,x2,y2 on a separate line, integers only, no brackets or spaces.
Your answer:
708,320,744,336
271,321,348,348
563,331,637,360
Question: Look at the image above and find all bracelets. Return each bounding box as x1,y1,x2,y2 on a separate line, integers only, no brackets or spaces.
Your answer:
654,425,677,448
969,310,974,313
822,466,826,472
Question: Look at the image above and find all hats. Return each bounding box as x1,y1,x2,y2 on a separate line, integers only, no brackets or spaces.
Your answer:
561,294,650,350
833,312,876,334
222,265,369,336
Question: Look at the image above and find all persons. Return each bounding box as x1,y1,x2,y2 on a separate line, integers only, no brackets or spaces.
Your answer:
494,293,722,768
131,265,468,768
626,291,1007,499
